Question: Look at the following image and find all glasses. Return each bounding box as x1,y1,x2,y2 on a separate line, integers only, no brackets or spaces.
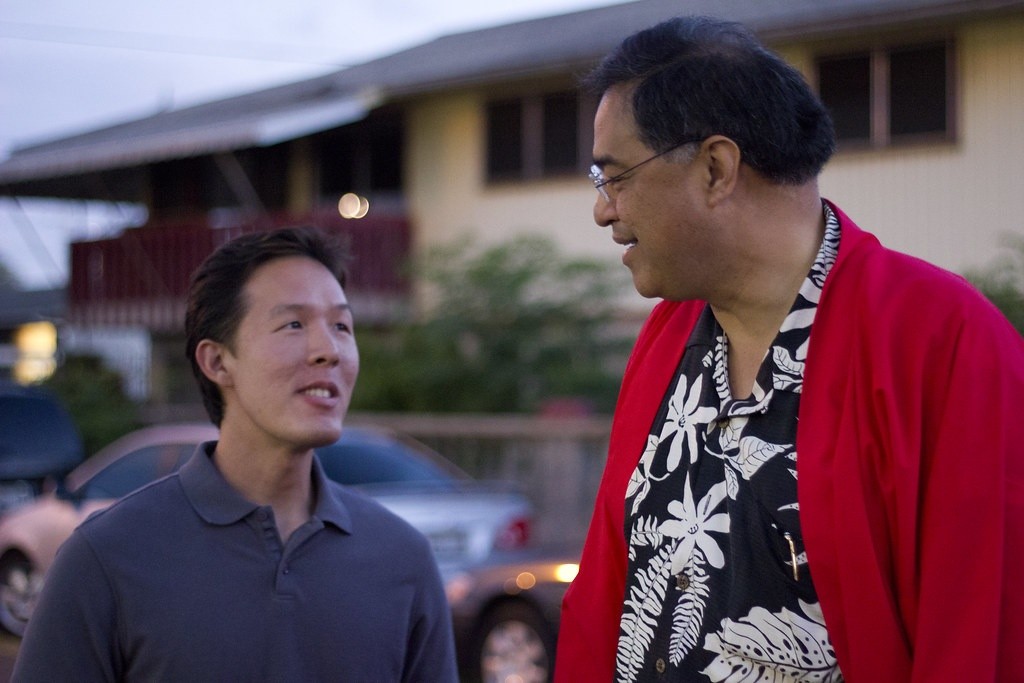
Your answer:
588,135,705,202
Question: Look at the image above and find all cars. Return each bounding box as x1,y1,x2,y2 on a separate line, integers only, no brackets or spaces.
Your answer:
444,541,601,683
0,422,540,645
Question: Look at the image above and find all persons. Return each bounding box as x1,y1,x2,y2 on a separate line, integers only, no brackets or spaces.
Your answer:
557,14,1024,683
9,224,461,682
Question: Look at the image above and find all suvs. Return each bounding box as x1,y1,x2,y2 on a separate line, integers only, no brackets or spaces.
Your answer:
0,385,83,517
